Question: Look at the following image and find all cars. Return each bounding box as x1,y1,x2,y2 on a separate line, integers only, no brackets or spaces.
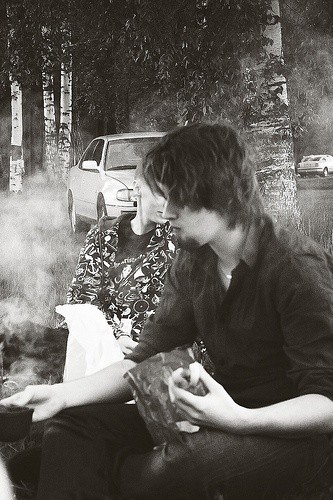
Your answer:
65,129,175,233
296,153,333,177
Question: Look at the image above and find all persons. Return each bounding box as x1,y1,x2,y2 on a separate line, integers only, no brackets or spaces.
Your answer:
0,124,333,500
0,153,203,385
